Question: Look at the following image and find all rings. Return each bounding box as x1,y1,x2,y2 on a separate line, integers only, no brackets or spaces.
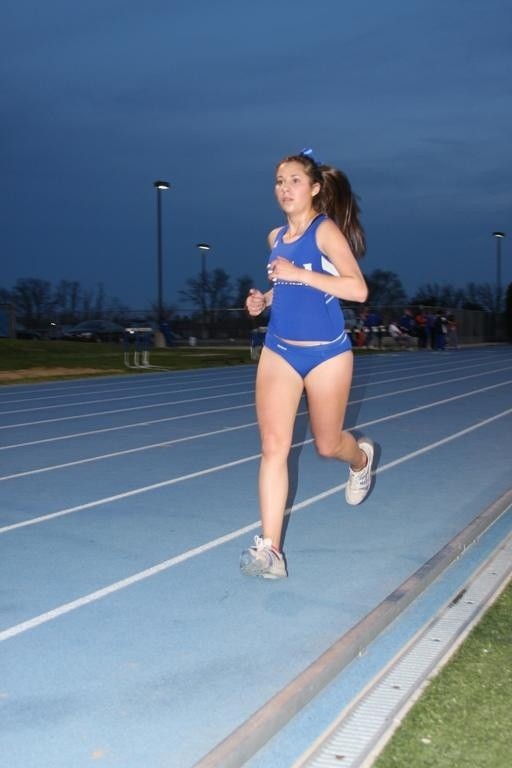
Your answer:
273,264,276,272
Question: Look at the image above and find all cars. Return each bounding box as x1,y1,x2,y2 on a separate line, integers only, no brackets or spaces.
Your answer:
65,316,126,343
14,320,40,341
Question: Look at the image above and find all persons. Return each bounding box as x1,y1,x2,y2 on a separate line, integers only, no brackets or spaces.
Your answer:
343,305,462,358
239,148,376,577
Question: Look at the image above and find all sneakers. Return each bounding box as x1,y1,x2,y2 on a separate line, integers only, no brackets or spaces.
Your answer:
236,540,293,584
343,434,379,509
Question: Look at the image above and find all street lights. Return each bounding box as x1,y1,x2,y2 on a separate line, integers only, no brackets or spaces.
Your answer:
154,180,170,333
491,232,506,338
196,243,212,339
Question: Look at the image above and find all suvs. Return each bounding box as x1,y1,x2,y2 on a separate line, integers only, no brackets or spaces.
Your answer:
253,306,368,345
